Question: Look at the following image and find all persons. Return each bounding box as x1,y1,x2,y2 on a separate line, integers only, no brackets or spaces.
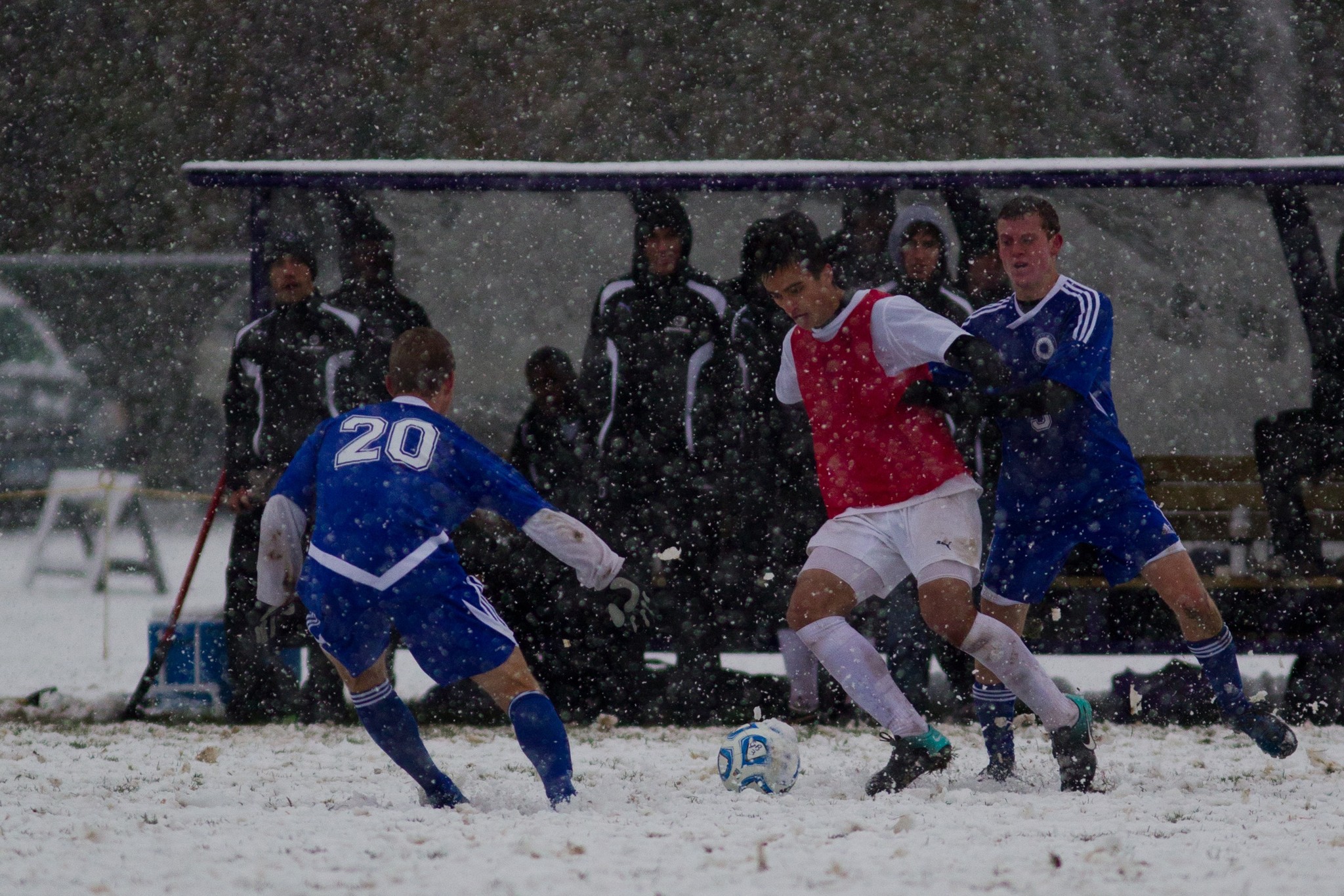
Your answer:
318,212,426,410
580,194,749,733
755,214,1097,794
713,214,1029,720
246,325,653,809
450,343,612,720
901,190,1297,786
222,238,372,727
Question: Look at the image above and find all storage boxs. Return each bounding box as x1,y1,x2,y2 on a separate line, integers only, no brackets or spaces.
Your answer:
148,618,303,725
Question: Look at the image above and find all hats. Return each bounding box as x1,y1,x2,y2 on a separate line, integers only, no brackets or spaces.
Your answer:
263,231,319,277
340,195,395,249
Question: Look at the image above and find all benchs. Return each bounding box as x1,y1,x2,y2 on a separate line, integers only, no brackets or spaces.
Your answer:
1020,435,1344,662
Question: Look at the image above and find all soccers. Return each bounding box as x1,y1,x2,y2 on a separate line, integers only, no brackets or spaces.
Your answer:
717,723,799,794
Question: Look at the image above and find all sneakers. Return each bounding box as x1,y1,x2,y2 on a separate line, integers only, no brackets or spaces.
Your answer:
1223,702,1297,759
1049,694,1097,792
781,709,819,727
418,788,469,808
975,762,1016,782
866,724,951,794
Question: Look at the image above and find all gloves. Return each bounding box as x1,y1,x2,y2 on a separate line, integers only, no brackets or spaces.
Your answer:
902,380,964,409
258,600,312,653
603,569,659,641
